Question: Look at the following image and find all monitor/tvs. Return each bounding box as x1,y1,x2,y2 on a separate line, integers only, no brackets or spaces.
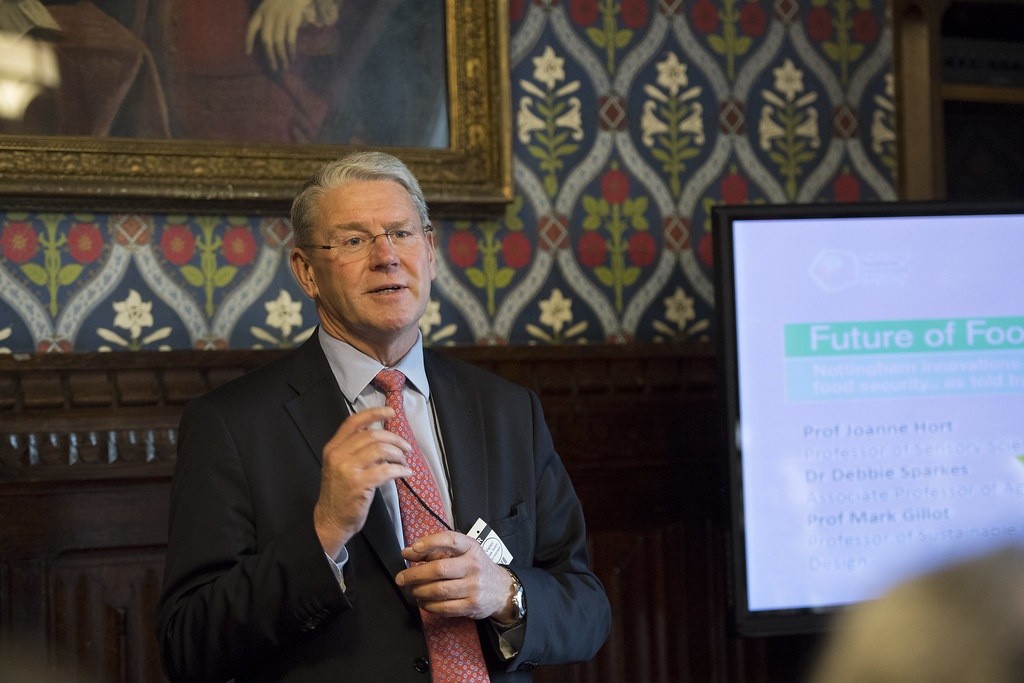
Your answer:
710,200,1024,635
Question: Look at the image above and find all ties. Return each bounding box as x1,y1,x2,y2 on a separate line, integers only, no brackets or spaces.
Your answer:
368,367,491,683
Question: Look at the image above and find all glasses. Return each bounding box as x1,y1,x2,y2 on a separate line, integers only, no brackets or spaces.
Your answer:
299,227,433,258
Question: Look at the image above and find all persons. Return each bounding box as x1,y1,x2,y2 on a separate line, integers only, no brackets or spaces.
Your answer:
154,151,611,683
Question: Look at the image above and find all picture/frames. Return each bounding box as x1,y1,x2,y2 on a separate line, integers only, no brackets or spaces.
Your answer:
0,0,514,218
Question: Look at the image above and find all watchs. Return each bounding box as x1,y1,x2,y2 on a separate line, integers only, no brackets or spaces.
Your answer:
491,565,527,629
